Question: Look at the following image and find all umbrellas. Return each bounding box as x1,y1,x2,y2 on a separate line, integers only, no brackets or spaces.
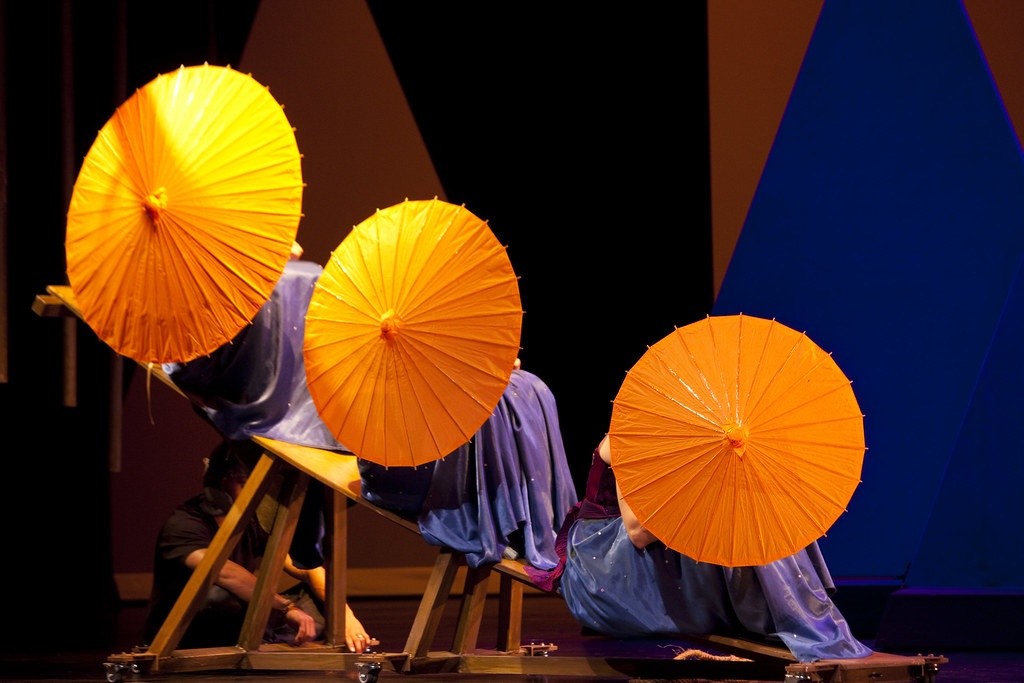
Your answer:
606,312,868,567
303,195,525,470
65,62,306,364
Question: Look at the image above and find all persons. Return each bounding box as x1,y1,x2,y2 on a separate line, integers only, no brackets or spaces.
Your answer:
523,432,873,664
356,359,578,573
141,439,370,653
161,242,355,568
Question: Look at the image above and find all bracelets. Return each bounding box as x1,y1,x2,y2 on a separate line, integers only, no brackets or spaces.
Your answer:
281,600,295,613
